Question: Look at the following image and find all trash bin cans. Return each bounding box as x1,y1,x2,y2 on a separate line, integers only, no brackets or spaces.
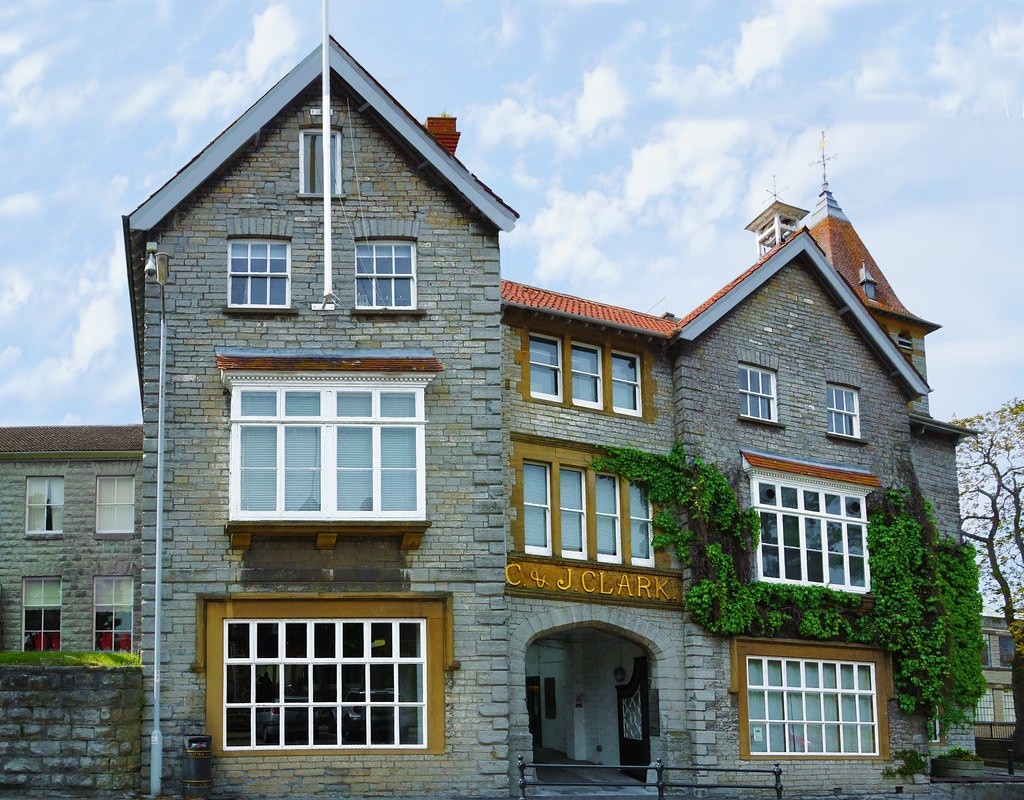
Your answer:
182,734,213,799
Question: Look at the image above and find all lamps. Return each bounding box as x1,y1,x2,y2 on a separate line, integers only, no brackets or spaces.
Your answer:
613,667,625,682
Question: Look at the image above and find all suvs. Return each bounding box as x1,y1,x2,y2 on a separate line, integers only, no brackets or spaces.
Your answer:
328,688,410,745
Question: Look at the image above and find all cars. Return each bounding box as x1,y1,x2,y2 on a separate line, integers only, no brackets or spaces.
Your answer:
751,723,831,751
257,696,320,743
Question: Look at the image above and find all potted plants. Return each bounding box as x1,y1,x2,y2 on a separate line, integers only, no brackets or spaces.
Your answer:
929,744,984,778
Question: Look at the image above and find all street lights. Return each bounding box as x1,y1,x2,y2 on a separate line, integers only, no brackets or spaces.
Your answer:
142,241,167,799
